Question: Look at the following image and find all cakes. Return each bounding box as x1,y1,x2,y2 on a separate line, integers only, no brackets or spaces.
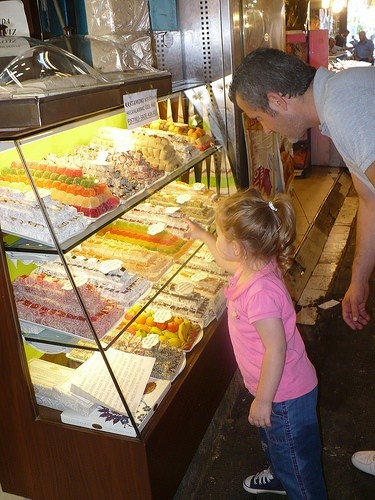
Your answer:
103,218,186,254
104,128,180,173
139,239,229,327
74,143,165,190
39,153,143,201
0,182,89,245
0,74,97,94
133,128,201,161
8,274,124,340
140,119,216,151
30,253,152,307
121,180,220,238
0,162,122,220
70,305,201,380
71,234,174,281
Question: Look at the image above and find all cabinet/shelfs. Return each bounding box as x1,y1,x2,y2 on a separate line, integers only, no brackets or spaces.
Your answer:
0,79,241,500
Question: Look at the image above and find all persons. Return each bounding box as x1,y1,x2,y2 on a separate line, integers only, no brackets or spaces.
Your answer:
228,46,375,475
329,30,374,65
180,187,328,500
